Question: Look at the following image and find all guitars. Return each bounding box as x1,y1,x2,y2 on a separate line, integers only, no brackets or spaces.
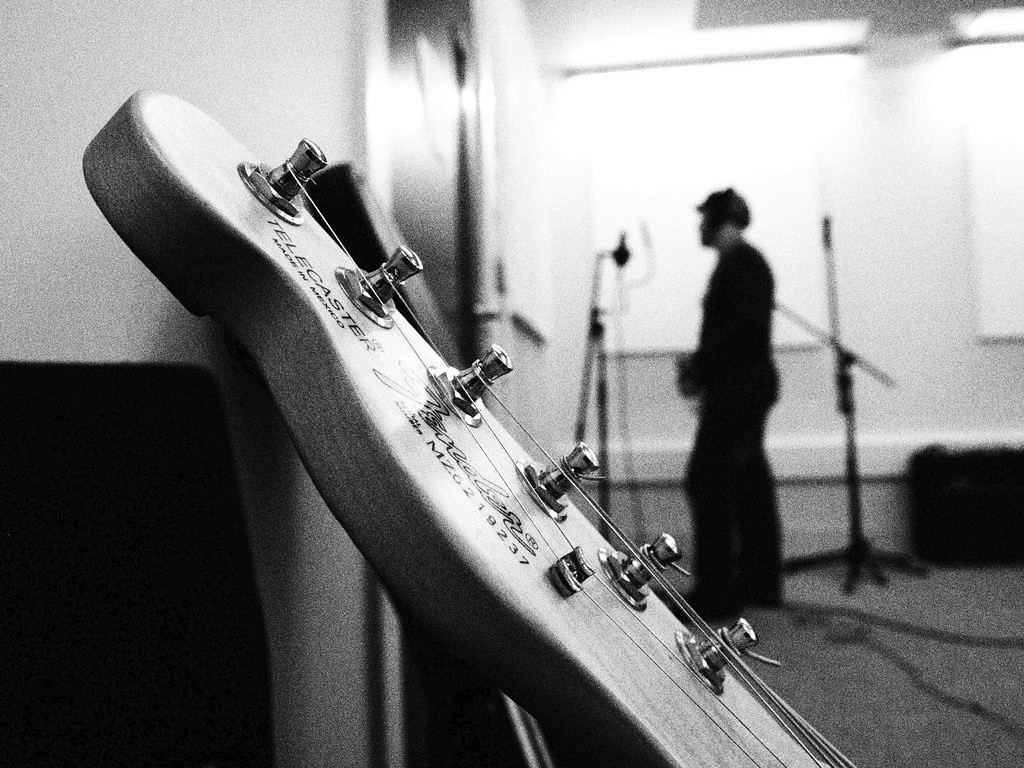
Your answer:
85,89,858,768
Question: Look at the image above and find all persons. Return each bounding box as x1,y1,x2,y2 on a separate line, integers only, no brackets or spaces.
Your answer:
667,189,787,619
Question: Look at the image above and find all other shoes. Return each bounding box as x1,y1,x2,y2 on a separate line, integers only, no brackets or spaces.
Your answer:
739,581,783,606
671,585,738,620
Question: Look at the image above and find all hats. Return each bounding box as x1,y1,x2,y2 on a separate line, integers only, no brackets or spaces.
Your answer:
699,189,751,229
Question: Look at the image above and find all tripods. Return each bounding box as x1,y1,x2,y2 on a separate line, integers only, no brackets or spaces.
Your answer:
772,214,924,593
